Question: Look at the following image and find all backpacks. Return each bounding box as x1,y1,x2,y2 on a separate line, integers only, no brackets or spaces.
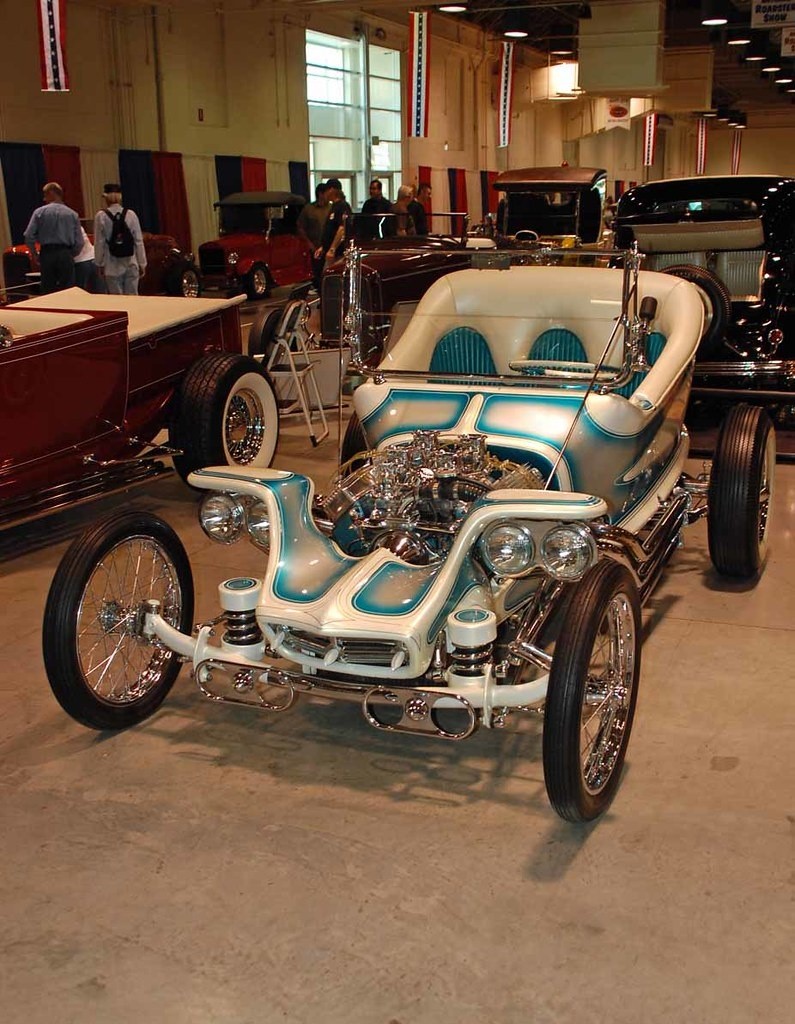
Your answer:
102,208,135,258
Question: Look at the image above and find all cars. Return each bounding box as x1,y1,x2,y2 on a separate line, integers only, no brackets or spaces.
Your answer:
42,244,777,825
198,189,320,300
612,176,795,431
323,160,610,364
0,218,282,558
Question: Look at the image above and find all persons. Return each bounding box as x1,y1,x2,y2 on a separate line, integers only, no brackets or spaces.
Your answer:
297,178,353,297
73,226,94,288
23,181,85,295
361,179,394,242
407,183,432,240
392,184,417,240
94,183,147,295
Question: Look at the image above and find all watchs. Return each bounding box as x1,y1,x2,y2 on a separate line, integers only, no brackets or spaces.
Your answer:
329,248,336,254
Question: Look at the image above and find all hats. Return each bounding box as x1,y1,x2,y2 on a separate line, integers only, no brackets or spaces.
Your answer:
103,184,122,192
325,179,341,189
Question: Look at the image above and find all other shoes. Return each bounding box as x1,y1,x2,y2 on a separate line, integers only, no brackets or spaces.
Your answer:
308,289,317,295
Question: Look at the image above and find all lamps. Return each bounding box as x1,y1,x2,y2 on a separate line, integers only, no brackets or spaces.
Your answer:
704,101,748,129
501,0,528,37
549,24,574,55
699,0,795,93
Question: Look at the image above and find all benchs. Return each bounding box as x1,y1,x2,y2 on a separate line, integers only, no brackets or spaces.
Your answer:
351,266,704,410
630,218,767,304
